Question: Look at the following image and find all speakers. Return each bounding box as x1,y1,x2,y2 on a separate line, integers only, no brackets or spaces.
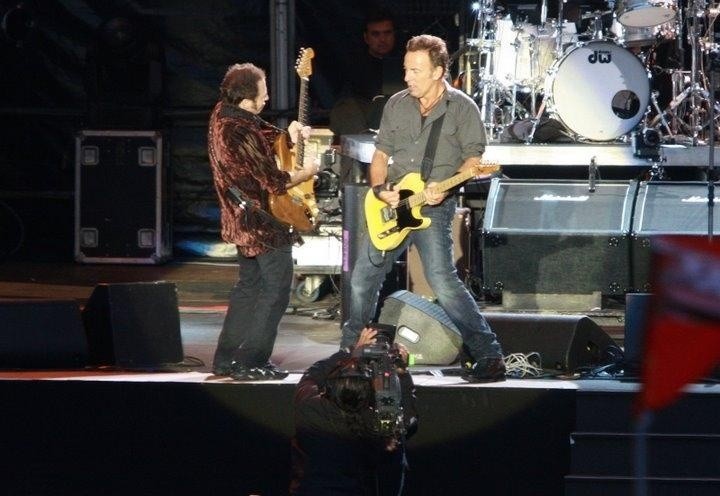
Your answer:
482,178,639,295
379,290,464,365
81,282,184,369
631,180,720,293
481,313,621,371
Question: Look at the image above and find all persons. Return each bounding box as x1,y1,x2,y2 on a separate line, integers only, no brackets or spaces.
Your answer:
330,10,409,136
288,327,421,496
206,60,317,381
341,34,508,383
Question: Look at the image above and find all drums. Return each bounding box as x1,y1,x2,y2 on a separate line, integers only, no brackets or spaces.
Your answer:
504,35,563,92
645,66,711,143
479,13,541,89
543,39,651,142
613,0,679,27
612,10,679,50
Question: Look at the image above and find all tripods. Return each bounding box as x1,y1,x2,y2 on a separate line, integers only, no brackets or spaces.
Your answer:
471,0,716,144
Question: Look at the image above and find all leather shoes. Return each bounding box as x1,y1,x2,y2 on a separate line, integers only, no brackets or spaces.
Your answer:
318,197,343,217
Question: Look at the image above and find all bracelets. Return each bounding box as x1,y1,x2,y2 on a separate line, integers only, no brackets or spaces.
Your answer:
373,181,394,195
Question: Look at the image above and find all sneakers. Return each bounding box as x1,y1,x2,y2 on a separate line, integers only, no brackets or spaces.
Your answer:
211,358,275,381
230,361,290,381
461,357,508,383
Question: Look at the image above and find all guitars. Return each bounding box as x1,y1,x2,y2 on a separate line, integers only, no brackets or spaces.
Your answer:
268,47,322,233
364,160,500,250
